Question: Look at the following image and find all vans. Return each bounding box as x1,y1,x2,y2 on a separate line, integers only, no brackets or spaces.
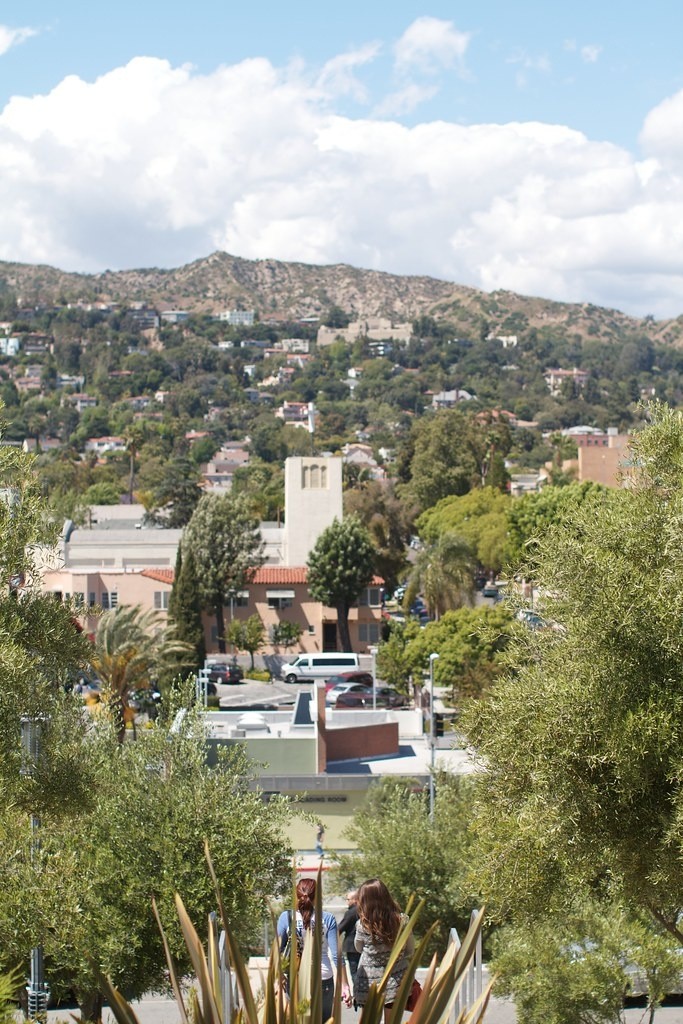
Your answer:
280,652,361,683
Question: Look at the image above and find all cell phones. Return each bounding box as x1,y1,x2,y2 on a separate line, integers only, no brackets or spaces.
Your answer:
343,993,350,1006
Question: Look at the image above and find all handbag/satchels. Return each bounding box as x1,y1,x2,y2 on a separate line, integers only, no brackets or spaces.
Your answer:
279,910,303,993
404,979,422,1012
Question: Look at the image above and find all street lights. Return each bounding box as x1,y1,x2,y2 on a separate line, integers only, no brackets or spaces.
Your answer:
370,649,378,710
428,653,440,821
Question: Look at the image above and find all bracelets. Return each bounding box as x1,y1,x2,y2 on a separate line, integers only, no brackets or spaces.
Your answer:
343,985,350,990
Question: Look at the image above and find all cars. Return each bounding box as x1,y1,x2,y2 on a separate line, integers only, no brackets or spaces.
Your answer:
324,670,409,709
396,581,432,629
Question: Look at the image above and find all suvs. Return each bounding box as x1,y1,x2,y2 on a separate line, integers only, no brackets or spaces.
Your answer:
207,661,244,685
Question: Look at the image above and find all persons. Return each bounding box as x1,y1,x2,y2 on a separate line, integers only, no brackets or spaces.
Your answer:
338,891,361,982
275,879,352,1024
354,879,416,1024
316,824,324,858
